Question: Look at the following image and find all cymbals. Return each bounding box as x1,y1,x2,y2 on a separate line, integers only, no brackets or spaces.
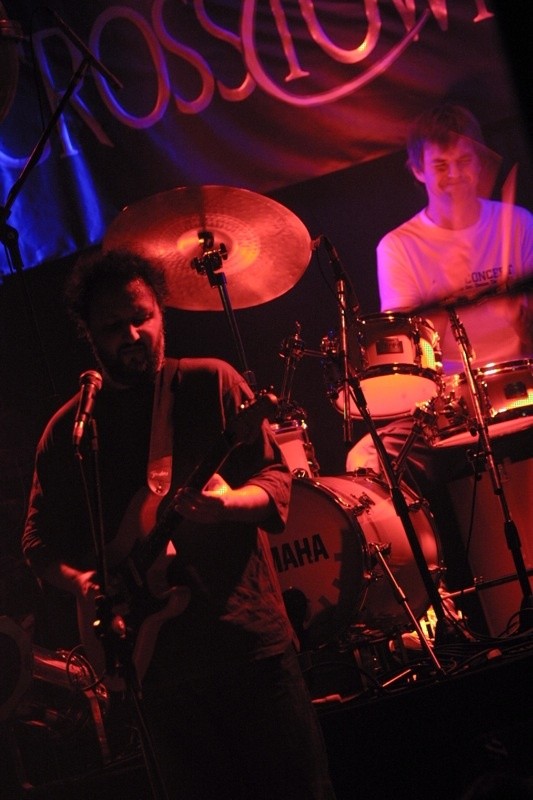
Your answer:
99,183,313,312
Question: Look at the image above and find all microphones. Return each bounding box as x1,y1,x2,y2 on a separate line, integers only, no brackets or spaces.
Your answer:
322,233,363,313
69,368,102,447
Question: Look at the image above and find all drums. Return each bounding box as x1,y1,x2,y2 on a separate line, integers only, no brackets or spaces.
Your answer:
324,304,452,422
422,359,532,448
255,397,319,496
257,463,443,680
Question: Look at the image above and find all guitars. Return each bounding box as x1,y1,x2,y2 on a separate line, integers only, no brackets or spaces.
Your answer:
59,378,282,679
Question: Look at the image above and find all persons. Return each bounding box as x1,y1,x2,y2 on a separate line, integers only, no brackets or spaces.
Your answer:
377,104,533,669
22,251,338,800
